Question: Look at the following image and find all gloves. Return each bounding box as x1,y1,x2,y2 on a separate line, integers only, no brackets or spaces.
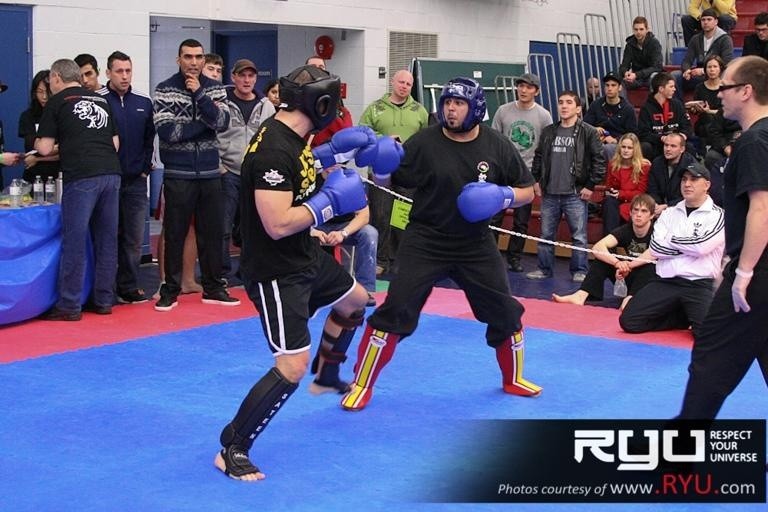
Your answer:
304,126,404,227
457,182,515,223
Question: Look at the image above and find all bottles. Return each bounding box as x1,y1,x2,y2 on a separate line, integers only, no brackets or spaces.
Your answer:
8,172,66,210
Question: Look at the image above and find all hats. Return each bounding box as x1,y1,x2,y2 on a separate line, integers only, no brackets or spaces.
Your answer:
603,71,621,84
515,73,540,89
678,163,710,181
233,59,258,75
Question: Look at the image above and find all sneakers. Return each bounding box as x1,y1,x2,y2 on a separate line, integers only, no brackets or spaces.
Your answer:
508,256,586,283
39,281,241,321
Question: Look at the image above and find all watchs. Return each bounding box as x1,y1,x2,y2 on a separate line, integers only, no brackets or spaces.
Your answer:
340,229,348,240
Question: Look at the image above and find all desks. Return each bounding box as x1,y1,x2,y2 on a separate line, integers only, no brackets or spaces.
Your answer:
0,188,96,325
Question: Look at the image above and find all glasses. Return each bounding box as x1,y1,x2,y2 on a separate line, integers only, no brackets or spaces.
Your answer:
719,82,755,91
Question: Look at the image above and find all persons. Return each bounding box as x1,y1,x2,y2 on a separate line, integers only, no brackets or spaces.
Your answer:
262,78,281,112
489,0,767,419
0,47,153,324
213,63,378,483
216,58,276,295
307,164,379,307
358,69,430,279
152,36,241,311
153,52,224,301
305,55,353,150
340,76,543,415
525,90,609,282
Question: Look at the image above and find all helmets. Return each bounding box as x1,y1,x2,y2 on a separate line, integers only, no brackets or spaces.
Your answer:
279,65,341,130
438,76,487,132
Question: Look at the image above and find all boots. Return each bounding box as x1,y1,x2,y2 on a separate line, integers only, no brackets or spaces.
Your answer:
340,324,400,411
496,328,543,397
